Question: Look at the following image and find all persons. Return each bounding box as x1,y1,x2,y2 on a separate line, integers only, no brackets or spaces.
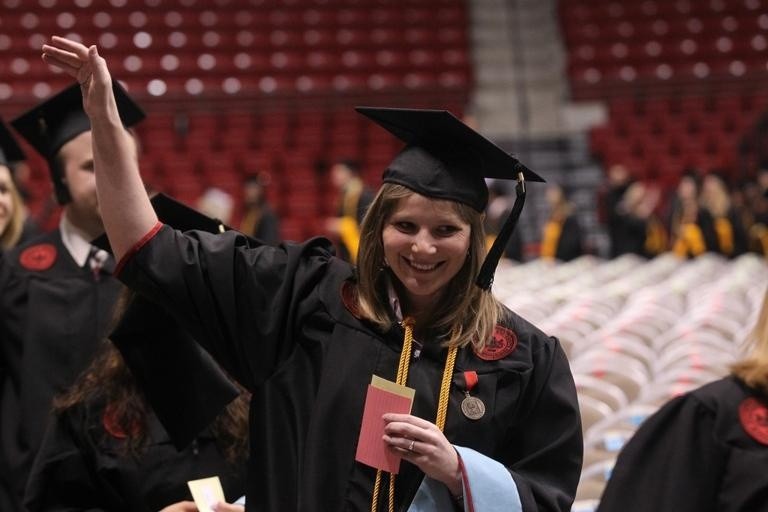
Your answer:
0,119,38,250
22,280,253,511
316,156,375,266
484,162,766,264
38,35,583,512
595,273,768,512
225,167,285,246
0,68,147,512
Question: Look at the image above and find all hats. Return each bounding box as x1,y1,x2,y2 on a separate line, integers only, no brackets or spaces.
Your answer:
353,106,546,291
1,77,146,167
88,192,272,255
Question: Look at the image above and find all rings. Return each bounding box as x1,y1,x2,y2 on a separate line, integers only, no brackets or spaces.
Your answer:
409,441,415,452
